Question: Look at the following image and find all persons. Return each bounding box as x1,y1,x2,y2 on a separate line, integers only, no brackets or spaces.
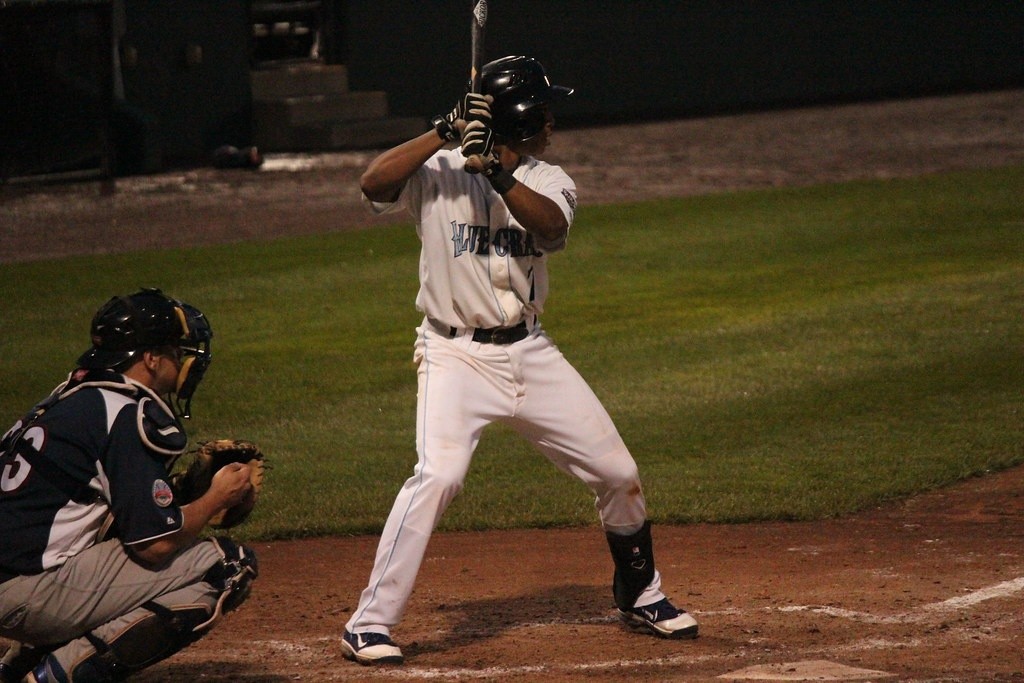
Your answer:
0,283,265,683
339,50,701,664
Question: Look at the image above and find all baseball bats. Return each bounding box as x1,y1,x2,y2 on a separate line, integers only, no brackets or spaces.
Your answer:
464,0,490,174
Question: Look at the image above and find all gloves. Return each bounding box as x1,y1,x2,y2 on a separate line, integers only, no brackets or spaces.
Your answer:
463,119,495,173
442,92,496,140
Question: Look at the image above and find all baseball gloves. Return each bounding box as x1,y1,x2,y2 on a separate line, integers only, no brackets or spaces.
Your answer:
185,440,267,530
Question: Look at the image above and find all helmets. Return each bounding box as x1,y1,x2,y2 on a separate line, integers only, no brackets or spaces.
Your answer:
71,288,211,412
476,51,573,145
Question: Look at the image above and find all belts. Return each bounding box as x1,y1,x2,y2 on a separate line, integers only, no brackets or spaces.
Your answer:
448,321,531,346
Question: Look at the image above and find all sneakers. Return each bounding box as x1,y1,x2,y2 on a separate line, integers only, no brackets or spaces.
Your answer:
343,629,404,665
621,596,701,638
